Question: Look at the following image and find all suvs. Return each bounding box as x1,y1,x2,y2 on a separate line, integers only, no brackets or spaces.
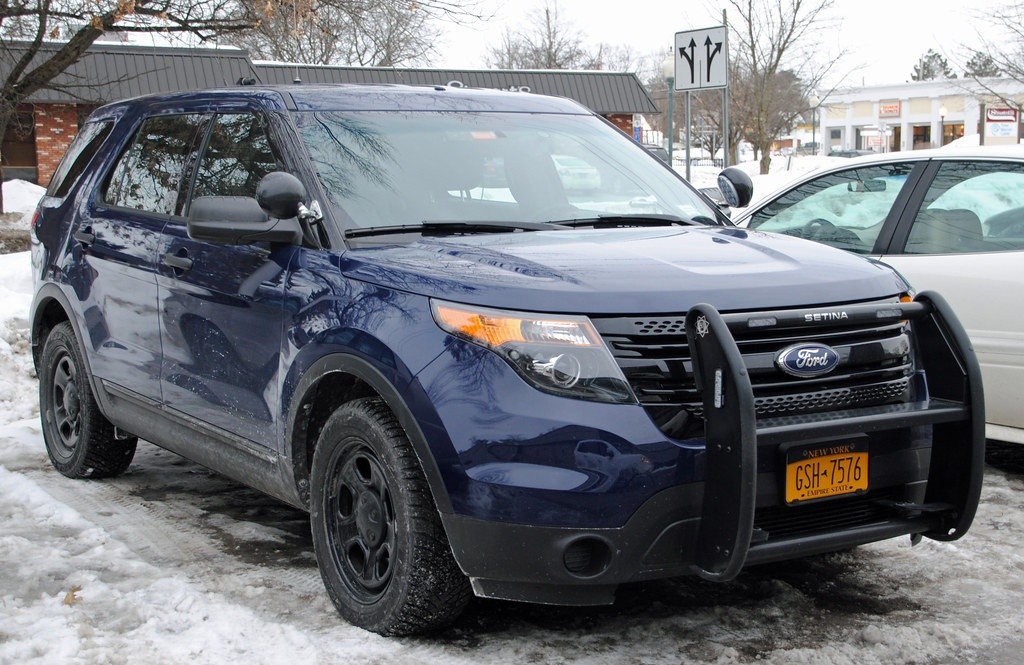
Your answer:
27,81,985,640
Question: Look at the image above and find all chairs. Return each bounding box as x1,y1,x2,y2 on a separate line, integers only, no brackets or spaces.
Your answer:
425,142,488,220
913,207,984,253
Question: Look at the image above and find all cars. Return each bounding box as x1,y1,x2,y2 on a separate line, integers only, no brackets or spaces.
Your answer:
728,142,1023,451
